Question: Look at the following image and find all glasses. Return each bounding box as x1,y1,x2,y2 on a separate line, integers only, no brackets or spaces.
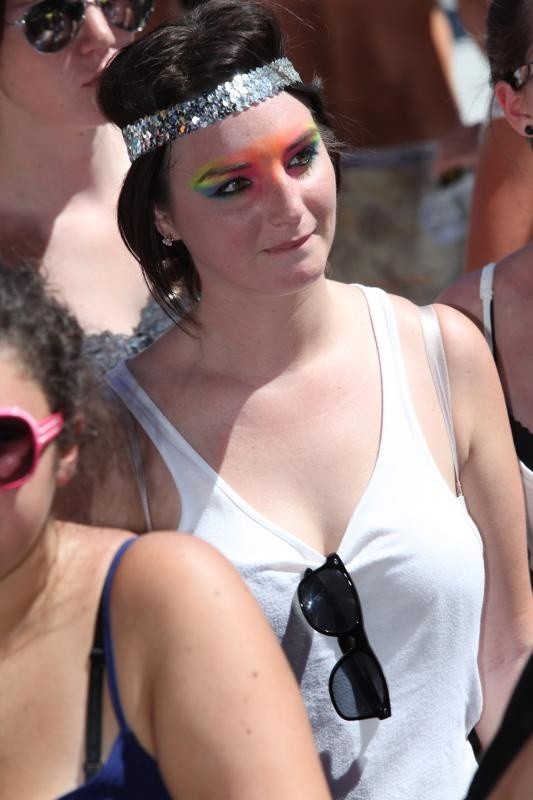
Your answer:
0,409,64,493
0,0,156,55
296,554,391,721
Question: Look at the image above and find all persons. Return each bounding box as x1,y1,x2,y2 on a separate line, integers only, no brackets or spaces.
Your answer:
430,0,533,587
465,650,533,800
0,251,336,800
0,0,198,394
52,0,533,800
